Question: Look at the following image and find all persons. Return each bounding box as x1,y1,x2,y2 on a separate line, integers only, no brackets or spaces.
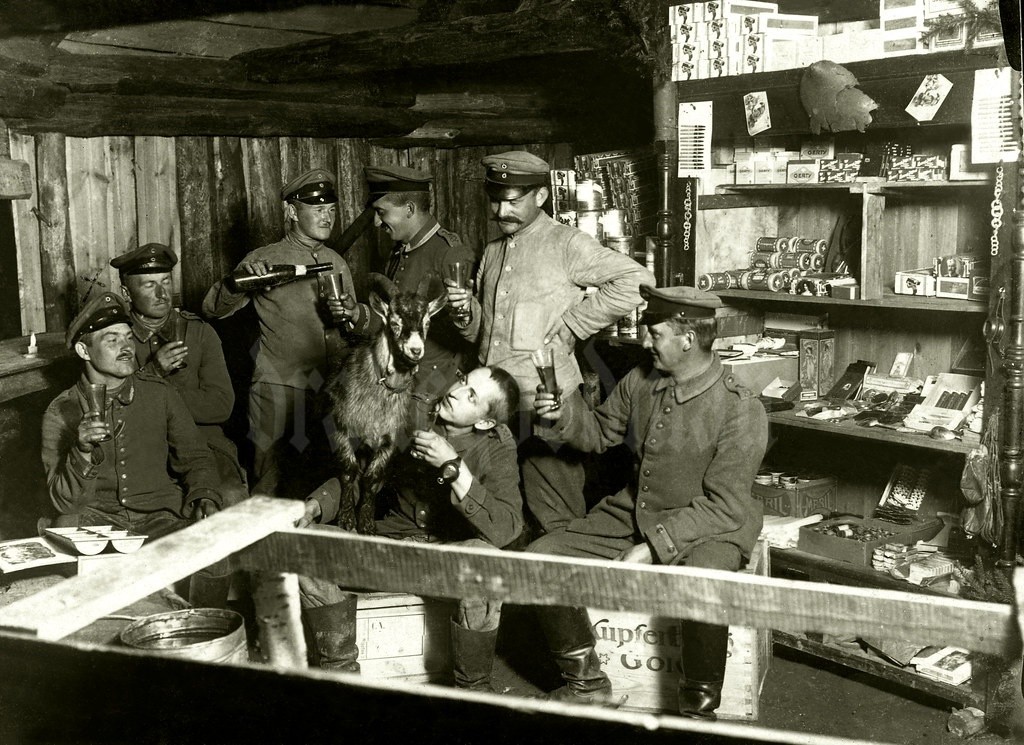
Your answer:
299,366,522,690
42,290,230,609
110,243,247,503
327,166,473,400
443,150,656,530
203,168,359,501
946,259,957,276
526,286,767,721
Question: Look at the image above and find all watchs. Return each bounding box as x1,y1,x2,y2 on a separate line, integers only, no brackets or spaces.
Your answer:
436,457,462,484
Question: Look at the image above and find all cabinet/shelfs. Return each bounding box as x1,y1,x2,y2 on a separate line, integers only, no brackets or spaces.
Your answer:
673,0,1024,714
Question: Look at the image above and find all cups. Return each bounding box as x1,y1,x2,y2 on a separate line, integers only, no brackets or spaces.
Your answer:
85,384,112,441
449,262,470,316
530,349,561,410
318,273,351,322
170,319,189,368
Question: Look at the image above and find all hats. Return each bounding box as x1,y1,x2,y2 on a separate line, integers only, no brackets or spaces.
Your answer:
363,166,433,194
65,292,129,349
282,167,339,205
637,284,723,324
110,243,178,275
482,151,549,200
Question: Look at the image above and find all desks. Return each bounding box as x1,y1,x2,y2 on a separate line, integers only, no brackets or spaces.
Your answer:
0,535,191,649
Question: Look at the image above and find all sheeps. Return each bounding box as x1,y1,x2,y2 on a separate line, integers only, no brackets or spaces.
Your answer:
312,270,450,534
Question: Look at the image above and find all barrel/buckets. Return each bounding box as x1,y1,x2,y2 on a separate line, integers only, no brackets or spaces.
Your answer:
120,608,249,664
575,172,638,337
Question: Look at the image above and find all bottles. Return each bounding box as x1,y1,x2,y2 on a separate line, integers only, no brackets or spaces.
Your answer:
232,262,333,291
411,400,441,460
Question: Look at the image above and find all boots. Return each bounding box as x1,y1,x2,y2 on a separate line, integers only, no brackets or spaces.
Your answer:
189,569,229,608
664,680,722,724
521,644,611,710
449,614,501,692
304,593,360,675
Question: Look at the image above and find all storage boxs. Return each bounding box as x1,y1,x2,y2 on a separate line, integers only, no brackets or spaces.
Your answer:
340,589,457,685
865,371,924,405
749,474,944,568
895,265,994,304
729,133,1000,183
585,533,775,722
668,0,1007,82
550,166,577,229
